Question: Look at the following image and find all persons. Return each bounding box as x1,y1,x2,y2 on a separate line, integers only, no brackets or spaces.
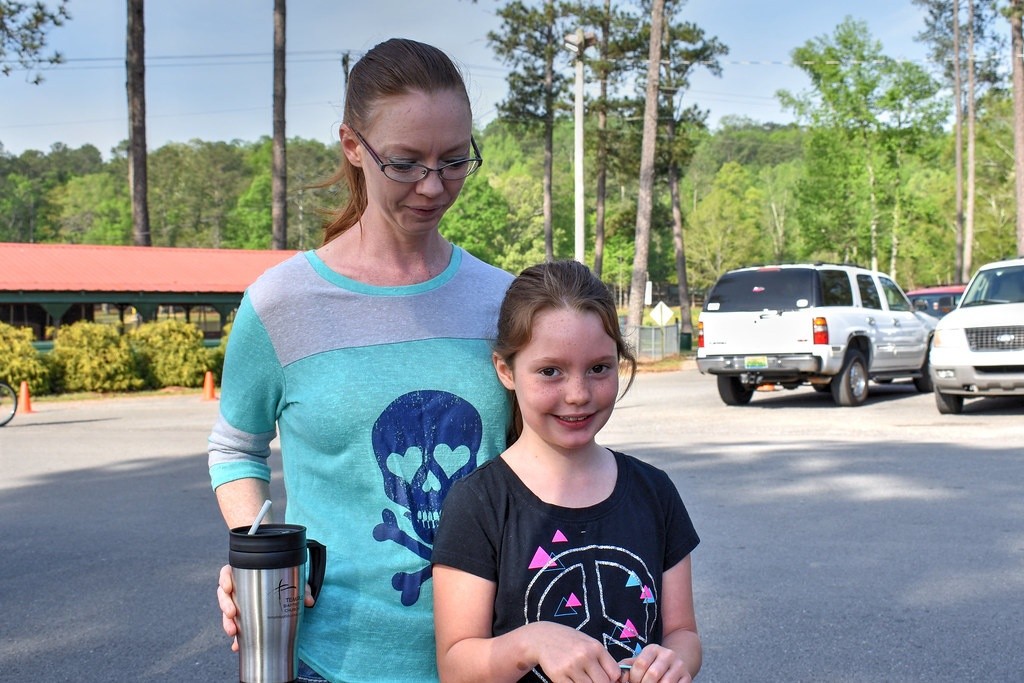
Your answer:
431,260,702,683
208,38,519,683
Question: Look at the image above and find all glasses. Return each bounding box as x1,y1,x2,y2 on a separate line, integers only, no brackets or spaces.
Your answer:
350,123,483,183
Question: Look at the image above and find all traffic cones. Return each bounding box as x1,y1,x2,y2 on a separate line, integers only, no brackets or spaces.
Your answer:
15,381,38,414
199,371,220,402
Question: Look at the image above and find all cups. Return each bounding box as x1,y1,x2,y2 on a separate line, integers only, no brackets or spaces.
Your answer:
229,524,326,683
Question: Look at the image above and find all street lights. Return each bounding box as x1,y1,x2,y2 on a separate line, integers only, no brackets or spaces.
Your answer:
562,27,597,264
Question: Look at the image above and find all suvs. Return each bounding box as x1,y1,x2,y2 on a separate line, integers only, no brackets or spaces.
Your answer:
695,261,940,406
905,256,1024,414
